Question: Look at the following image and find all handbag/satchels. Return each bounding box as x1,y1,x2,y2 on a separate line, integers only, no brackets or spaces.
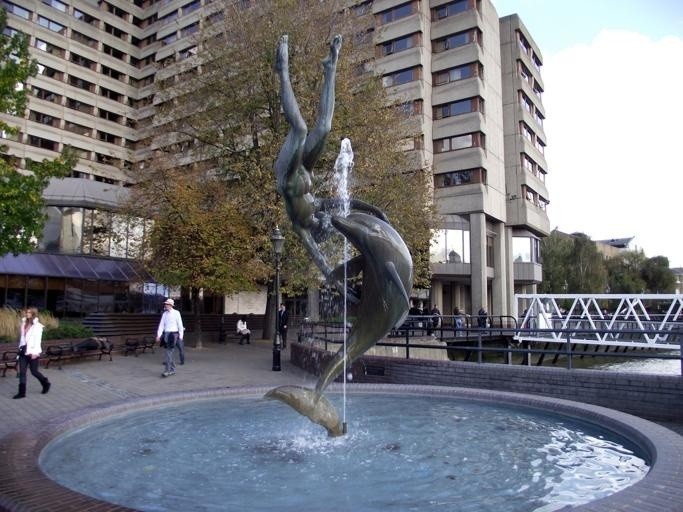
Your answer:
17,345,27,356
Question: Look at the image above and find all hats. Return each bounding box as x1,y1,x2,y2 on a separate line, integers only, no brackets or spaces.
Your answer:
163,298,175,307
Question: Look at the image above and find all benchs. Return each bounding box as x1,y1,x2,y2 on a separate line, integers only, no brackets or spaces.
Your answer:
225,325,252,345
43,337,113,370
0,343,21,378
125,336,155,357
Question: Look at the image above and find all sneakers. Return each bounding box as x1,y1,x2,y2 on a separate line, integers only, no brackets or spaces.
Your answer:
162,371,176,377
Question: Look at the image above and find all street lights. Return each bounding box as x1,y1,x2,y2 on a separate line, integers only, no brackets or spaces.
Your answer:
219,243,232,344
270,225,286,372
561,279,569,318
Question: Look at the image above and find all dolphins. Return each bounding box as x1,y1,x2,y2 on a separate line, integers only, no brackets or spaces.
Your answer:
263,212,415,437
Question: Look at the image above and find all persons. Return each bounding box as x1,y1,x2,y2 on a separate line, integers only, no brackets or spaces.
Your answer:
279,303,289,349
236,315,251,345
155,298,184,378
454,307,463,336
479,308,487,334
275,34,390,307
161,308,185,364
431,305,440,331
13,307,51,399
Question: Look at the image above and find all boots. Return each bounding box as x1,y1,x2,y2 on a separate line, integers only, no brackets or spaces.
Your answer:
13,382,26,399
40,377,51,394
